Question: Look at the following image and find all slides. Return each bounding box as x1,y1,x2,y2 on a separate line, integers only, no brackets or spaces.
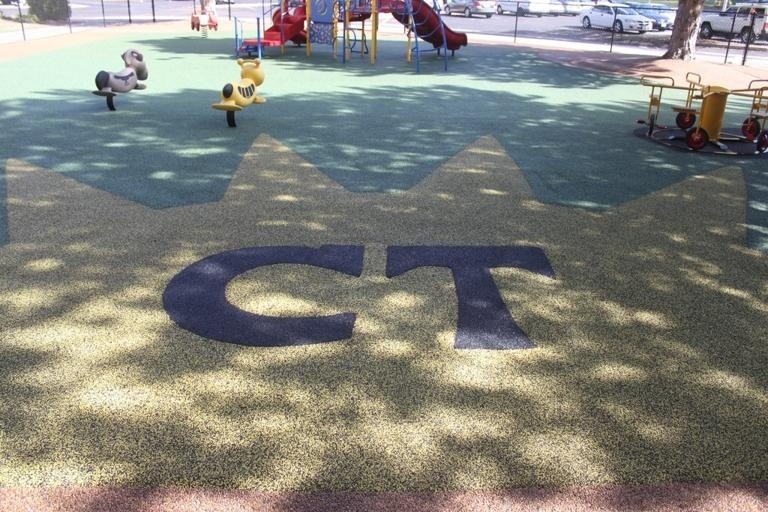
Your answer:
391,0,467,50
